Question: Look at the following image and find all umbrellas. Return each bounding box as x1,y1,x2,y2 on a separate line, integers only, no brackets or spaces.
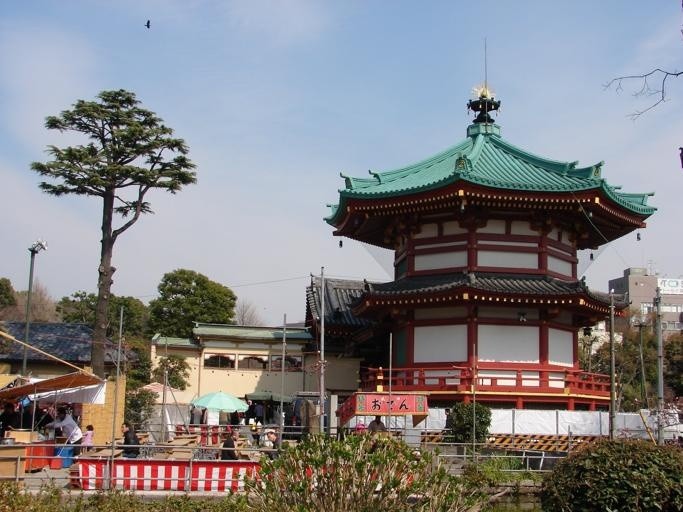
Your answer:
192,389,248,416
244,390,293,425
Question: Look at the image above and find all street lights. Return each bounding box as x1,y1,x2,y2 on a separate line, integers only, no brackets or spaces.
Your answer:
21,235,50,379
148,331,168,445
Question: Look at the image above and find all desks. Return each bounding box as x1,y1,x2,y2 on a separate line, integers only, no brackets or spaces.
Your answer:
89,431,304,459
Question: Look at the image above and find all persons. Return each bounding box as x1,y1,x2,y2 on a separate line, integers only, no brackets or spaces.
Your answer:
367,416,385,432
81,424,94,446
444,409,453,429
253,401,263,424
262,431,280,461
0,404,20,437
354,419,364,431
29,404,53,430
220,431,239,460
44,408,83,457
115,422,140,458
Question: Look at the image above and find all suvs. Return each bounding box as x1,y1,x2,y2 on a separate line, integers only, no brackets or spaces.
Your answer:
285,393,331,438
